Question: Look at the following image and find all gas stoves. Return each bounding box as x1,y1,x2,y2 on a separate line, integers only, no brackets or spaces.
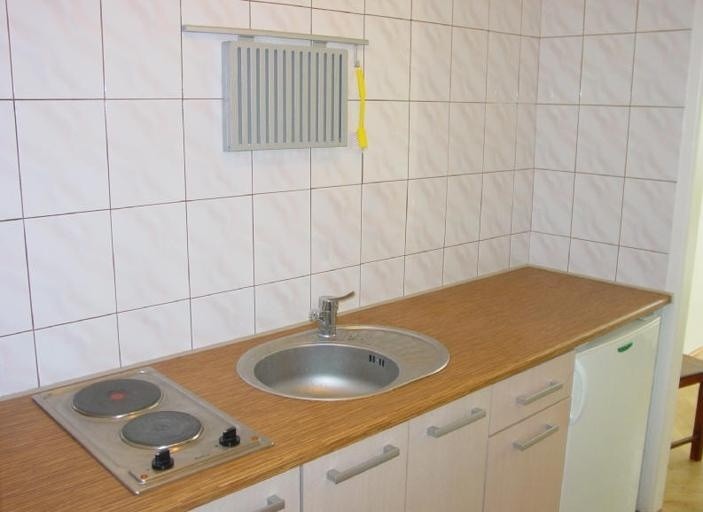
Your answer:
32,365,273,498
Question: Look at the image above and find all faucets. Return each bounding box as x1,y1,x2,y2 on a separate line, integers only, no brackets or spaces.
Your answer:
308,291,356,342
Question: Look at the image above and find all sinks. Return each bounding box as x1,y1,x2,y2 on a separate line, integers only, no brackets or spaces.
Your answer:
235,322,451,403
